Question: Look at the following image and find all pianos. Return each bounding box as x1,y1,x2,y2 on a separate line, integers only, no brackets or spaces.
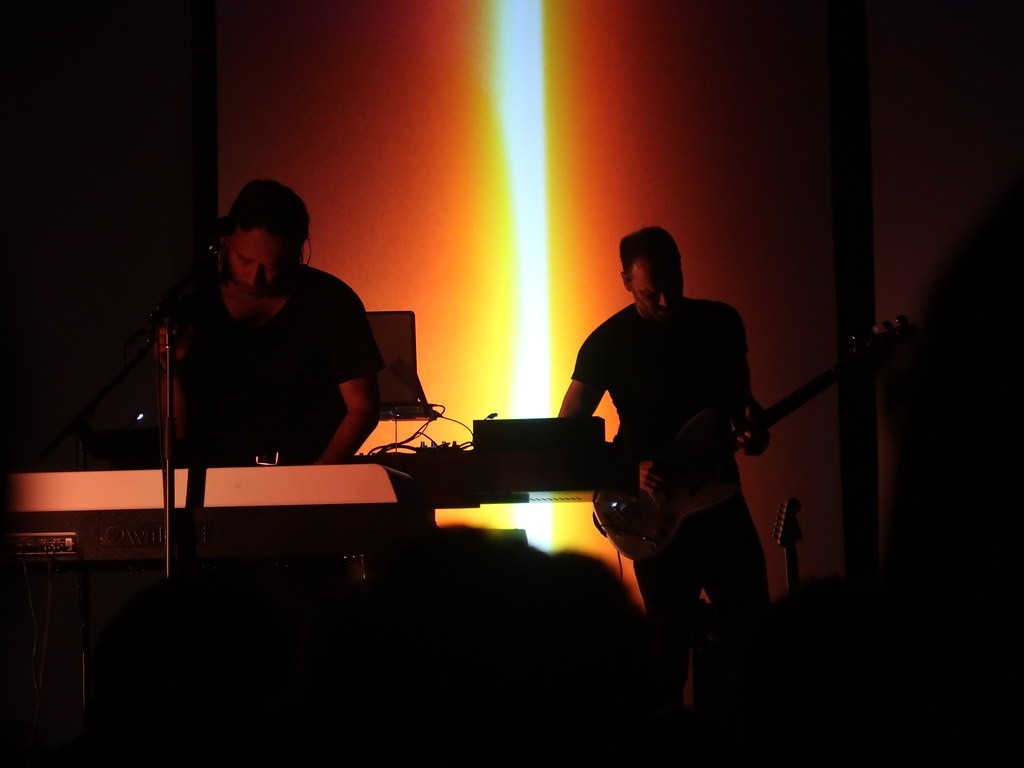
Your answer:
0,456,434,565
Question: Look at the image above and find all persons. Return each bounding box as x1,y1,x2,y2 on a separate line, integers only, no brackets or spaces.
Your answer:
558,227,770,702
885,174,1024,768
153,179,382,467
77,527,1024,768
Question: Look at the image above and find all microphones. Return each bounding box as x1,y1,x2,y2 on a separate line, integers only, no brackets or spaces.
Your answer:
204,216,237,238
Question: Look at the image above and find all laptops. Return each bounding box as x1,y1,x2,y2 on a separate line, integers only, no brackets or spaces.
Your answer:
365,310,427,407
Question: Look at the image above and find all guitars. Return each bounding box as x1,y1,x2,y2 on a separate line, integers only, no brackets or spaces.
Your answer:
593,315,917,563
773,496,806,592
